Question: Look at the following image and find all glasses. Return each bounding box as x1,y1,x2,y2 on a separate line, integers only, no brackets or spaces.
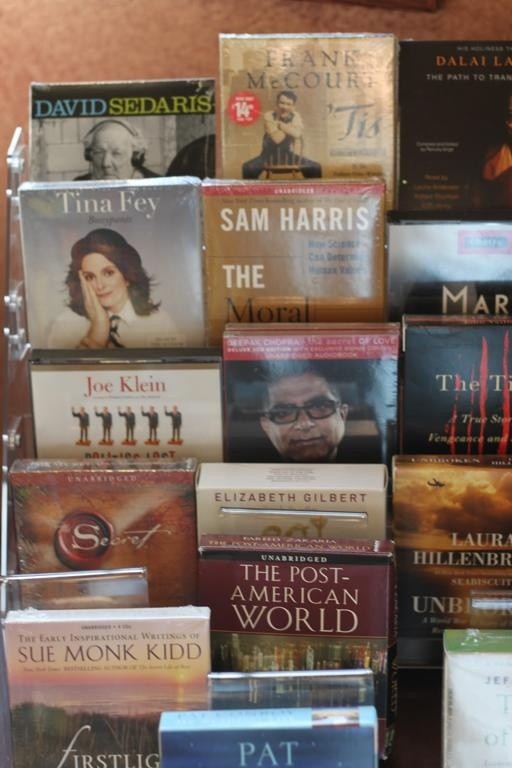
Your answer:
262,399,341,425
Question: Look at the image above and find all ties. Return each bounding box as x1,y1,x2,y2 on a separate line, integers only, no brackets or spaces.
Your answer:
109,314,125,348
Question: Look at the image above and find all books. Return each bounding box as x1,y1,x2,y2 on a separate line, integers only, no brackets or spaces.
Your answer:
0,31,511,767
